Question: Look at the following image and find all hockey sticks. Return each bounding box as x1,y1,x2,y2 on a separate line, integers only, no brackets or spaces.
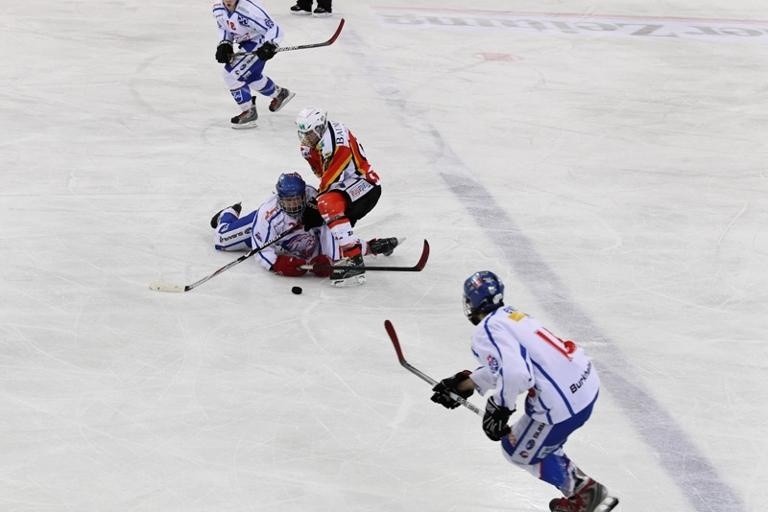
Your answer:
384,320,485,418
231,18,345,57
149,223,302,294
298,239,429,271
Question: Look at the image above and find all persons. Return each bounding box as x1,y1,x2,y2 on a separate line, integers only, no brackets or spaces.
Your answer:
210,1,290,124
296,105,398,279
427,269,609,512
208,170,340,281
289,0,333,13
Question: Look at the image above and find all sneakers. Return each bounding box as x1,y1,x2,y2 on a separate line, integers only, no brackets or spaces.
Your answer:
549,477,607,511
367,237,398,256
211,203,241,228
330,243,365,279
231,107,258,124
291,4,312,12
269,87,289,112
314,6,332,13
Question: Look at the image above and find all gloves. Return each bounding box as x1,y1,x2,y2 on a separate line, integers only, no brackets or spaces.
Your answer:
272,255,308,276
257,41,279,60
482,396,516,441
430,370,475,409
215,40,233,64
310,255,334,278
300,197,325,232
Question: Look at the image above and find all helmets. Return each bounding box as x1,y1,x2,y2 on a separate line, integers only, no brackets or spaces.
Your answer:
276,172,306,216
296,106,328,147
463,271,504,325
